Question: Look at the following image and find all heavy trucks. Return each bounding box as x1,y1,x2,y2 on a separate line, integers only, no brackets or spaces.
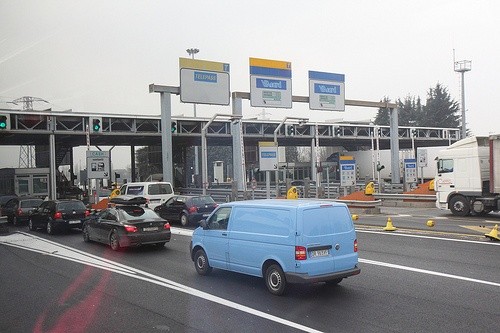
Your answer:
433,134,500,218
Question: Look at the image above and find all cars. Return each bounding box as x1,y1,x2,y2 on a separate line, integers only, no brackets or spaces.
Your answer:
0,185,121,222
154,193,221,227
81,205,172,252
28,198,90,235
7,197,44,227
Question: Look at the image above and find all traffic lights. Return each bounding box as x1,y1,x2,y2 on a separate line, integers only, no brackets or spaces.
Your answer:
93,118,100,131
377,164,385,172
170,120,176,134
0,114,7,129
252,167,260,174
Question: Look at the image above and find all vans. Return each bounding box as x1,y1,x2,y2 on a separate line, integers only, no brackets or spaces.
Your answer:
190,198,361,297
114,182,175,210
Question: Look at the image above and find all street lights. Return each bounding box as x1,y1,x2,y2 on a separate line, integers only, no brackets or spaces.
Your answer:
186,47,201,118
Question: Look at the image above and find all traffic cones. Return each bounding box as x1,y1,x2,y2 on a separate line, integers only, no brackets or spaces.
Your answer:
383,215,398,231
484,224,500,241
